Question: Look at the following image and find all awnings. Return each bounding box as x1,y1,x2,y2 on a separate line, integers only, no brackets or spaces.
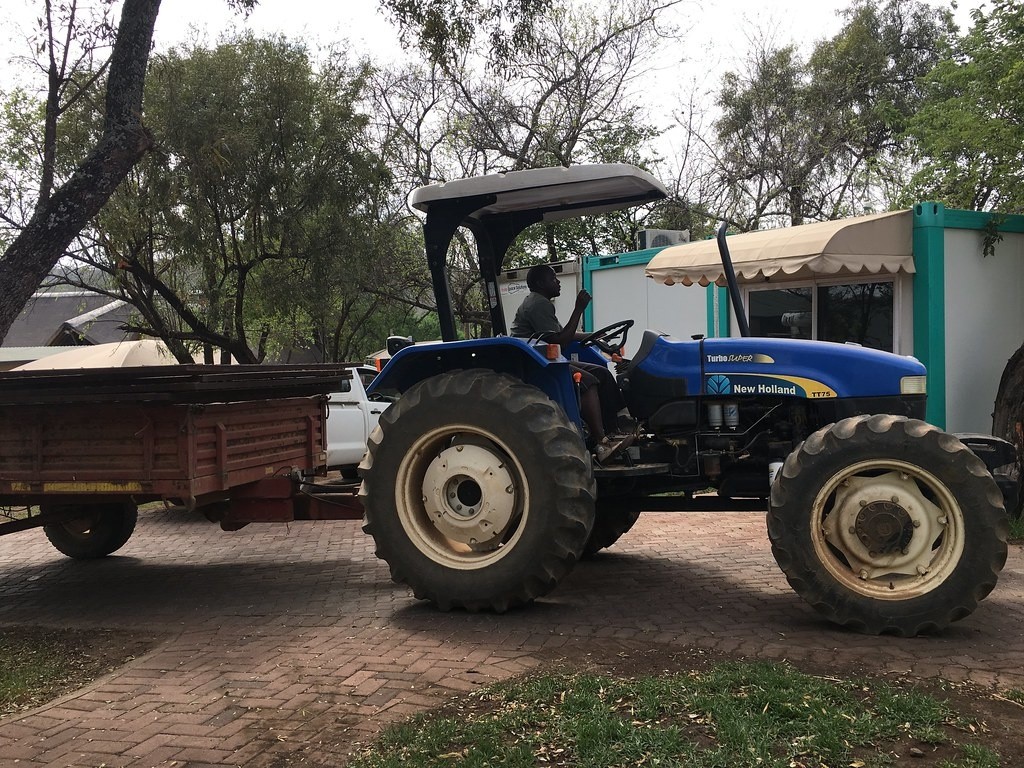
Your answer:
645,210,917,287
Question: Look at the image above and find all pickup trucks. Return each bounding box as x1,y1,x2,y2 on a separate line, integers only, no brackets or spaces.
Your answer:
313,365,399,482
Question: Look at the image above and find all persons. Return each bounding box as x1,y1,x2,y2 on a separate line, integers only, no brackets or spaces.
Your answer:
509,265,637,464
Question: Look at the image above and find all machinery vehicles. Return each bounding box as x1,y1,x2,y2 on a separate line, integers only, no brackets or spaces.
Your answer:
1,161,1009,637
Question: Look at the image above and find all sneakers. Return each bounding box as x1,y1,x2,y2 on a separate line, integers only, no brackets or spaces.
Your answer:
595,434,635,466
605,428,637,442
